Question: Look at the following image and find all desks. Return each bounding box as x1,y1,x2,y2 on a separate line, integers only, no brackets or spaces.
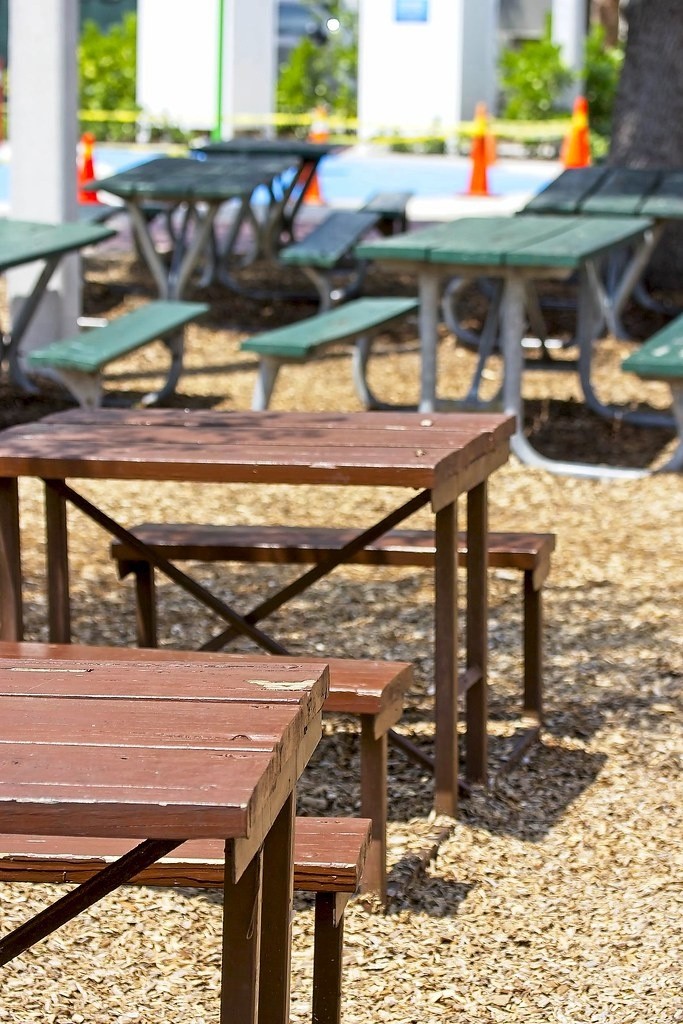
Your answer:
0,220,209,415
437,163,683,361
0,658,372,1024
239,215,683,482
0,407,552,915
159,140,408,298
76,155,379,348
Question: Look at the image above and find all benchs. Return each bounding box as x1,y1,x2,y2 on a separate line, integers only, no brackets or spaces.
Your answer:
0,188,683,1024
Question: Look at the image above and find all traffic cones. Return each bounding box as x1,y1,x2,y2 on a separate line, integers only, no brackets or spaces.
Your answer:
76,130,98,203
294,104,327,205
463,97,501,198
560,97,592,171
